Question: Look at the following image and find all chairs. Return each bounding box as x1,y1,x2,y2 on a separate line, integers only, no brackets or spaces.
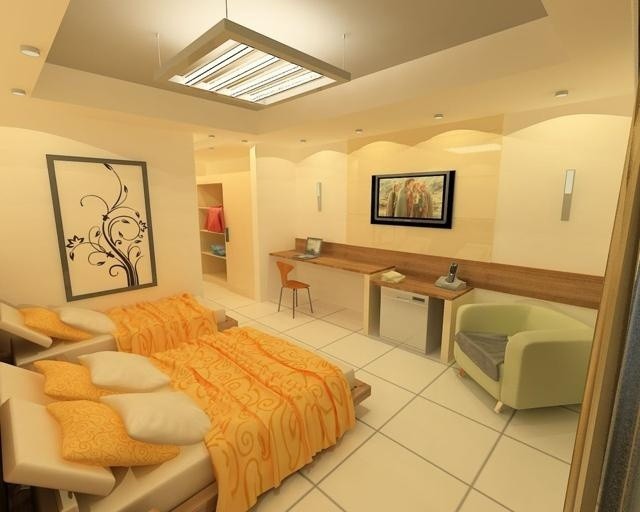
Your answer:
452,302,594,415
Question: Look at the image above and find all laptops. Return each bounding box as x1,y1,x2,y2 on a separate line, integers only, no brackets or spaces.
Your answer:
293,237,323,259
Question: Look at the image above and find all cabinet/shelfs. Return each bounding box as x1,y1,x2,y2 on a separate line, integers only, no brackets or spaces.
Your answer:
196,171,255,300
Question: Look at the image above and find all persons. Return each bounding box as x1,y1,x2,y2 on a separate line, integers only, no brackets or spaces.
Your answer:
385,179,433,219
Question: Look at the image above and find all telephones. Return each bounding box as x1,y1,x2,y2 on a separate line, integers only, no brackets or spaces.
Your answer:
435,262,467,291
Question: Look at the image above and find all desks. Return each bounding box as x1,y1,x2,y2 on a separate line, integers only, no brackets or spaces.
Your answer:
268,248,474,362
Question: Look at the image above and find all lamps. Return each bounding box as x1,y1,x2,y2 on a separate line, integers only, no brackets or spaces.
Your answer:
148,1,352,113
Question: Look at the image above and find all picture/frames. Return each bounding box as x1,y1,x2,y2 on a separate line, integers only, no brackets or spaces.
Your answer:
46,153,158,302
